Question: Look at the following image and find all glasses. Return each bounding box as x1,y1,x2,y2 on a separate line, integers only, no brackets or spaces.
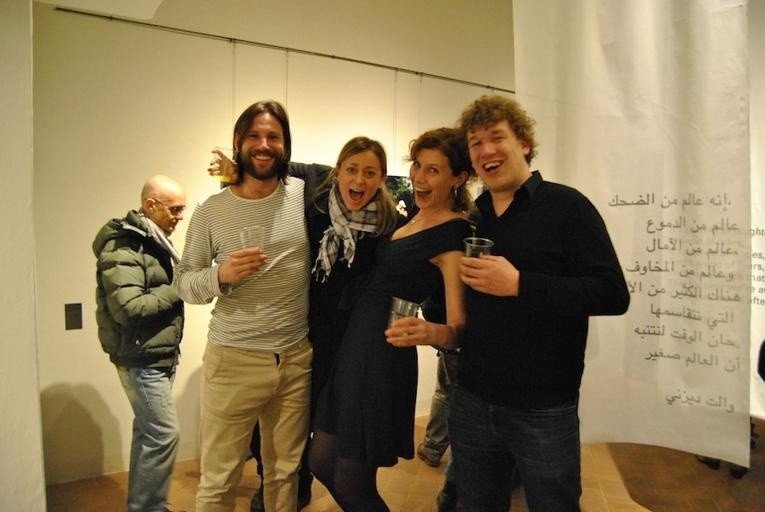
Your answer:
153,198,185,215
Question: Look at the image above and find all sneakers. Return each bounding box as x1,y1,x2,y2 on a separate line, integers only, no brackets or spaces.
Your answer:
417,442,440,468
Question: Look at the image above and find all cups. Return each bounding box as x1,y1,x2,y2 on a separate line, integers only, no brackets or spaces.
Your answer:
240,224,265,256
463,236,493,259
388,296,420,341
213,146,238,182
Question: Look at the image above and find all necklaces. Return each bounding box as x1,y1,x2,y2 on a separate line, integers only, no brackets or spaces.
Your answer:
411,207,448,225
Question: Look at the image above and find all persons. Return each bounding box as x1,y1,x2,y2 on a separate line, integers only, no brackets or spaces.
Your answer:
172,99,314,511
207,134,408,512
304,126,469,512
448,91,629,512
92,174,185,511
695,454,745,480
415,355,451,467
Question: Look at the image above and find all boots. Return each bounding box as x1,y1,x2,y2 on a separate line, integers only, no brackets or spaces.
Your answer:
251,467,314,512
